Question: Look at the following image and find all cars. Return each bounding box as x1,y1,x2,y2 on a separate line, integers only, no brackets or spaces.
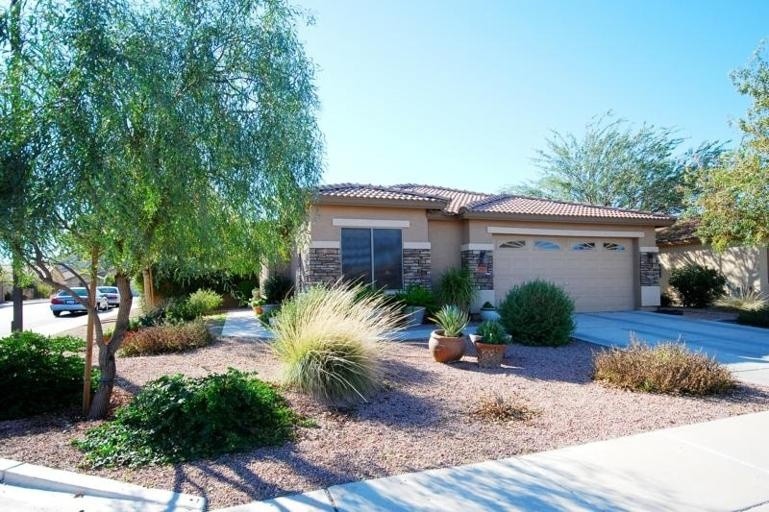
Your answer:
50,287,108,316
97,285,120,307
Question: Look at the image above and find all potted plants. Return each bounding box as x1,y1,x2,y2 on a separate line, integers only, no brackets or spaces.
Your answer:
252,268,512,408
